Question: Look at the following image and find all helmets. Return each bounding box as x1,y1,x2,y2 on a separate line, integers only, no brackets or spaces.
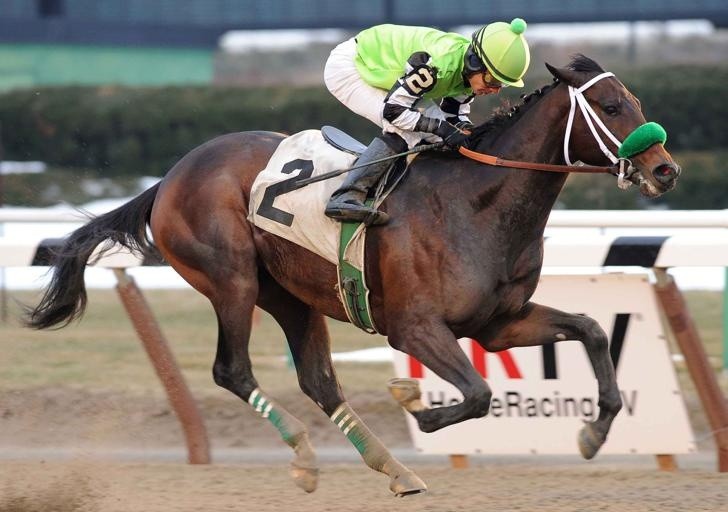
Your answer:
470,18,531,88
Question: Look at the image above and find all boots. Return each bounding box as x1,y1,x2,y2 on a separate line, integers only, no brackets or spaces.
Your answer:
325,137,399,225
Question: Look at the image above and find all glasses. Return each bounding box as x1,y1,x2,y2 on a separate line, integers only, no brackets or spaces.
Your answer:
482,71,509,89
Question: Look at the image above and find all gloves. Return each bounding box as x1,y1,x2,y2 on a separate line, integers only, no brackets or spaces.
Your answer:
433,121,471,151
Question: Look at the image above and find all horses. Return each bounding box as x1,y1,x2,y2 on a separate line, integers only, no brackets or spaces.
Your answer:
12,52,682,499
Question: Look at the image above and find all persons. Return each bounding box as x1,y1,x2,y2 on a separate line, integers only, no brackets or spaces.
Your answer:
323,15,531,223
